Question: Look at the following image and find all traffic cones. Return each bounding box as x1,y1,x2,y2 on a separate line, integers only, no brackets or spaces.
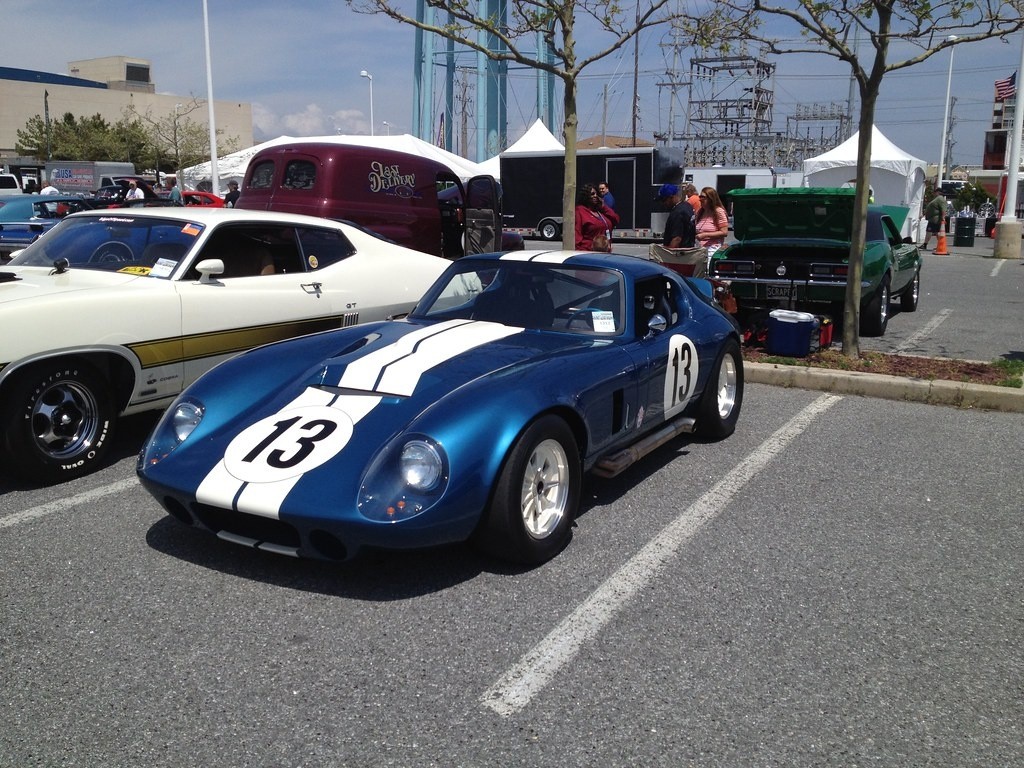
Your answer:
932,218,952,256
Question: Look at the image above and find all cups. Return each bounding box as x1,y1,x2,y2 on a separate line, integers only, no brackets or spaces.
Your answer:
621,232,627,237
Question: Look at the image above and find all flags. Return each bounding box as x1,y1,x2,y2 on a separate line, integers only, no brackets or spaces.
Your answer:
994,71,1017,101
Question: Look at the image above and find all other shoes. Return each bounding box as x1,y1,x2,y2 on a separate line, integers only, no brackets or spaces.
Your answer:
917,245,927,250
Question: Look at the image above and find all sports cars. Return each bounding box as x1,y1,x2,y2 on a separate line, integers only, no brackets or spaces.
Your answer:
134,248,745,561
708,187,924,336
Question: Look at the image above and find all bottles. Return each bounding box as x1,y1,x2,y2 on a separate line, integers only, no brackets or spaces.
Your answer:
634,229,639,237
643,230,649,237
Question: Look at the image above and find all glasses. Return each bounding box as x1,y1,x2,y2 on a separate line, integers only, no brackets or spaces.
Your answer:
591,192,599,196
698,195,707,199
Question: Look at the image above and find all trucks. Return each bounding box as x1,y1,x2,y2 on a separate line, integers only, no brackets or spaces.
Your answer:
500,145,686,242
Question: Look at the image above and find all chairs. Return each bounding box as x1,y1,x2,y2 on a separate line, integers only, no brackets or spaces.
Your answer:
471,270,553,329
586,277,671,334
138,242,188,267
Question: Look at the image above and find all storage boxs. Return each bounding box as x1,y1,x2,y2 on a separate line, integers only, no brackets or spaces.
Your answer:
765,309,814,358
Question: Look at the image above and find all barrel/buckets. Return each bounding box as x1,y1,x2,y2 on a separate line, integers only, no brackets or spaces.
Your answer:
952,218,976,247
985,217,998,237
766,309,833,357
933,216,950,232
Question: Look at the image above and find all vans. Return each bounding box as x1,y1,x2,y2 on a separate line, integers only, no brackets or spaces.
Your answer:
941,180,976,193
232,141,499,262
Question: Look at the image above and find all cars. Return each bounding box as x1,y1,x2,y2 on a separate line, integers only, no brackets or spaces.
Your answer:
0,159,229,273
0,205,486,483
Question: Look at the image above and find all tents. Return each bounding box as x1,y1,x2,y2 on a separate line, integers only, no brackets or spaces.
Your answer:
803,122,928,244
175,134,490,200
478,118,566,185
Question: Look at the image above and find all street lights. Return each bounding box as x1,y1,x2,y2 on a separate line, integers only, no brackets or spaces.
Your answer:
359,68,375,137
935,34,960,190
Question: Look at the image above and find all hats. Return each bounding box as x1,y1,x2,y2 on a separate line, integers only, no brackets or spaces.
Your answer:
226,180,238,185
934,188,943,195
652,184,679,201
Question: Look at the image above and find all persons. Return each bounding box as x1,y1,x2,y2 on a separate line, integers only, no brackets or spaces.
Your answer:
37,180,62,219
124,180,145,208
868,189,875,203
153,177,181,203
224,181,241,209
652,183,728,278
919,188,948,251
575,182,619,253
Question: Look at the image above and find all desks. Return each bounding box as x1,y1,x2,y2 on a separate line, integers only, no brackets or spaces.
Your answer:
945,216,998,237
